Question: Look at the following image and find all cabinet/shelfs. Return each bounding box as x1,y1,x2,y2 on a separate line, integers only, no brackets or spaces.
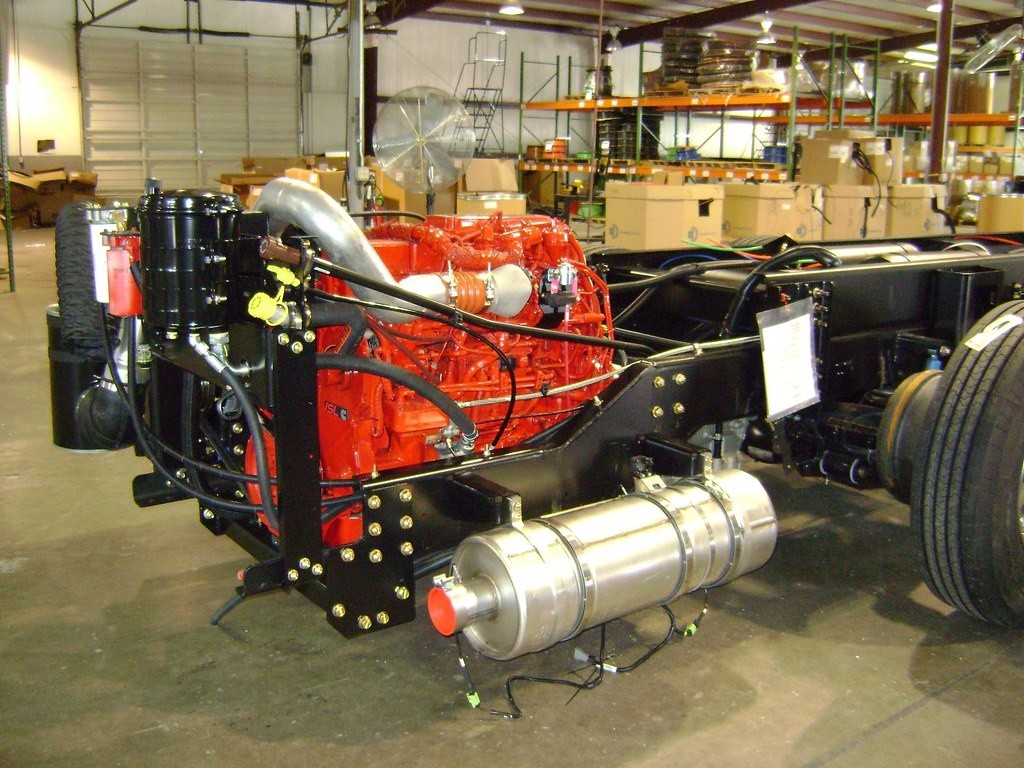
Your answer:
517,26,1024,245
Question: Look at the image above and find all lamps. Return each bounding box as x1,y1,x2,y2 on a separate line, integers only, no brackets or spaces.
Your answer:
757,19,777,45
605,25,624,54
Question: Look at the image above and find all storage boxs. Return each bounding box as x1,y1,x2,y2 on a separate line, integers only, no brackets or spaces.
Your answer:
799,137,865,186
955,155,969,174
969,157,983,174
373,167,427,223
427,182,457,216
522,169,563,207
719,182,824,244
888,184,948,240
0,166,108,230
863,137,904,184
814,129,876,140
455,158,519,194
822,184,889,243
457,194,527,217
220,154,346,210
983,156,999,175
999,156,1013,174
603,182,724,248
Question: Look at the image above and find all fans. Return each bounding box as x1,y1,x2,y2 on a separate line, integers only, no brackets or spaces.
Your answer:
947,22,1022,75
370,84,477,219
